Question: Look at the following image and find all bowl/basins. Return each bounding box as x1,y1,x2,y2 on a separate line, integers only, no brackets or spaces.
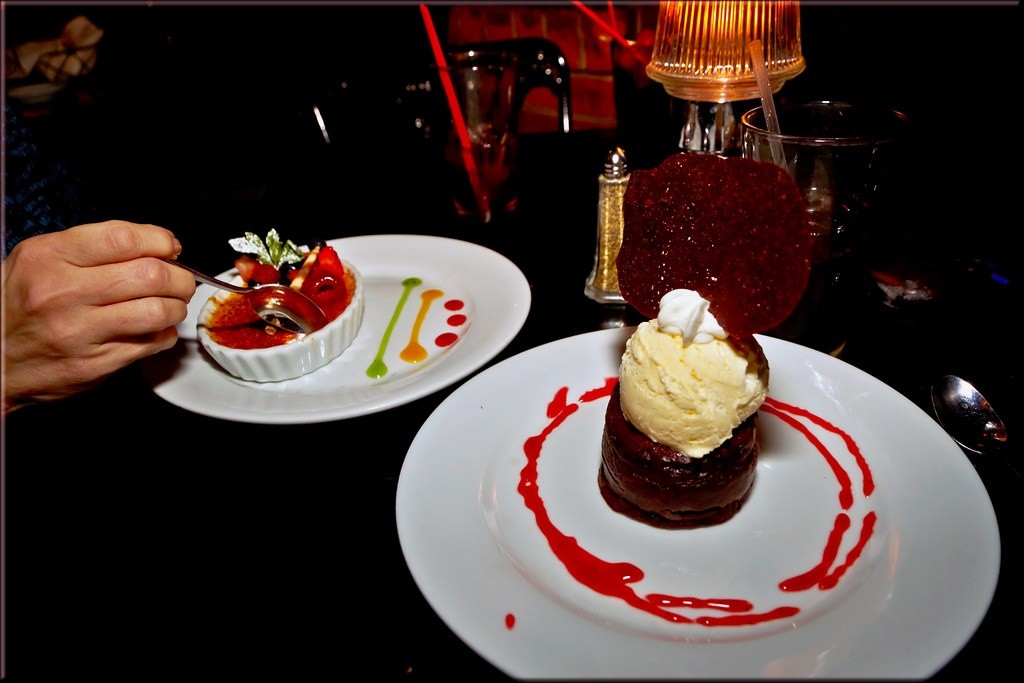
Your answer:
197,258,363,383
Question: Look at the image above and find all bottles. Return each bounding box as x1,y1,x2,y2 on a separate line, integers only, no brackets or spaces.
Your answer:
584,146,631,306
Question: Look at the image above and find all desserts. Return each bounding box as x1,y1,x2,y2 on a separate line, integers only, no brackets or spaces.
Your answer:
600,151,815,522
195,228,363,382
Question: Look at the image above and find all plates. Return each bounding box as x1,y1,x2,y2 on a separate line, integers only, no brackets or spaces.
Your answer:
142,235,532,424
10,85,64,101
396,327,1000,682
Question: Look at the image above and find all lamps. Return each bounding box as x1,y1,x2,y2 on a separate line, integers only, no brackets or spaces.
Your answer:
645,5,808,172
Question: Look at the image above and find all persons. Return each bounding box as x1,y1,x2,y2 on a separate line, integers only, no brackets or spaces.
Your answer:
6,217,196,415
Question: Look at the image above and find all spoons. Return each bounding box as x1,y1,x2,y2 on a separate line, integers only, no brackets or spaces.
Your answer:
931,374,1024,488
167,257,326,332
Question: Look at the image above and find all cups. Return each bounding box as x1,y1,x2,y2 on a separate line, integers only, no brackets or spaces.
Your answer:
739,100,911,286
428,51,526,213
